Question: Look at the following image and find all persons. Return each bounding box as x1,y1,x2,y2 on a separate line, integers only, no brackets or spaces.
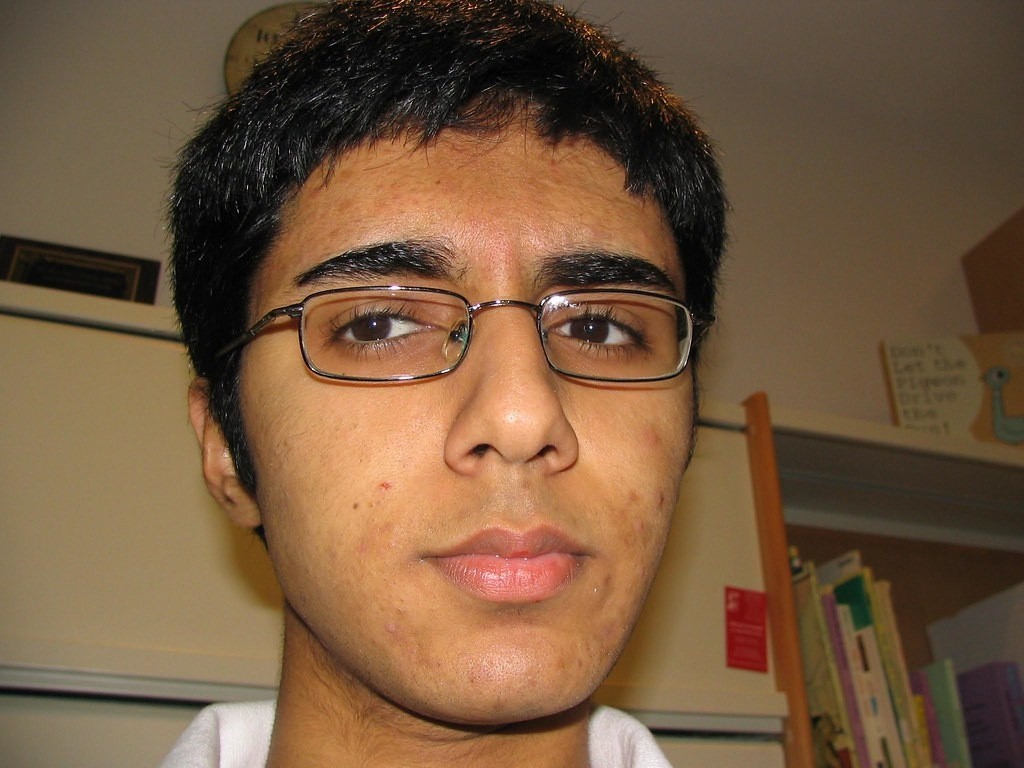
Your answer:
149,0,726,768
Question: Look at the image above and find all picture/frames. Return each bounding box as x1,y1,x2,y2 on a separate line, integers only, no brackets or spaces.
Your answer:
0,233,162,306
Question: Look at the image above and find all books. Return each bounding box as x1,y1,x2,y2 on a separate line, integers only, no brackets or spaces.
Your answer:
879,331,1023,446
790,545,1024,768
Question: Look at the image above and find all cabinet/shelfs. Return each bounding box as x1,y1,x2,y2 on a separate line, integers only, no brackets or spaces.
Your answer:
741,394,1024,768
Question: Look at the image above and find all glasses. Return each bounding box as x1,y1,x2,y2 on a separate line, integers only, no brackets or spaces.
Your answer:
213,285,716,381
